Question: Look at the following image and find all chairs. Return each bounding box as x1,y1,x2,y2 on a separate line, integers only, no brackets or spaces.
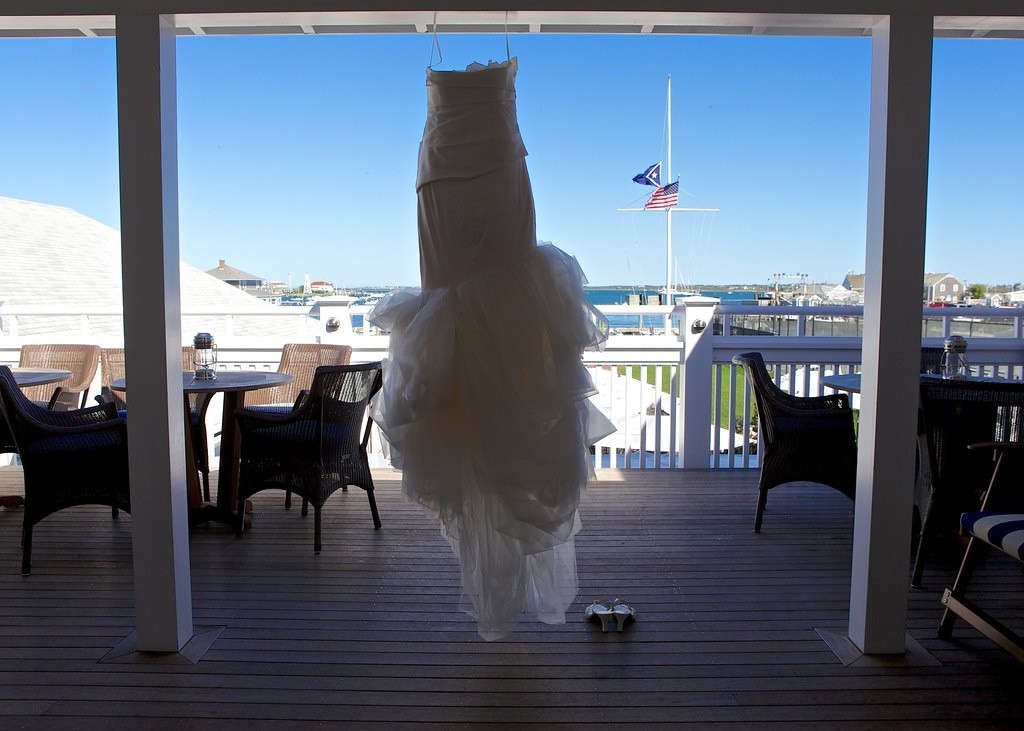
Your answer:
731,350,1024,680
0,344,387,578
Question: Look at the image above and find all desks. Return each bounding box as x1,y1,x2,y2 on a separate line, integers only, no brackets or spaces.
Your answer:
110,371,296,541
821,374,1024,412
10,368,73,387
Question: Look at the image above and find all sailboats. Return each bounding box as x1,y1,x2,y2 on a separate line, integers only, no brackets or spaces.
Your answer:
577,73,776,345
658,255,701,296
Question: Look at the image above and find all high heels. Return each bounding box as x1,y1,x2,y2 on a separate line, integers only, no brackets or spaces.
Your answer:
584,596,637,633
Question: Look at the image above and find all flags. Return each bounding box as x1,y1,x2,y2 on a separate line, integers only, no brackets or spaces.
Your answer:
644,182,679,209
632,162,660,187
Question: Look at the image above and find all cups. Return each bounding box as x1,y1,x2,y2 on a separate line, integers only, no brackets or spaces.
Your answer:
944,336,966,381
194,333,214,377
